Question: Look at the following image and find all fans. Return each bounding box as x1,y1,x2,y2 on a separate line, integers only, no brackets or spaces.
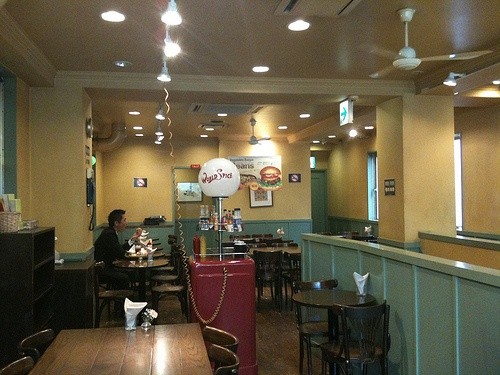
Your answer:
359,8,488,80
226,118,272,145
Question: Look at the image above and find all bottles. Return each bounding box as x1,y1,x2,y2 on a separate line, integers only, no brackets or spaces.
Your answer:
199,204,240,231
193,232,206,258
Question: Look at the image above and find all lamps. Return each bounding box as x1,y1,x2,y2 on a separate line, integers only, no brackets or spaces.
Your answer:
441,72,465,87
153,0,182,145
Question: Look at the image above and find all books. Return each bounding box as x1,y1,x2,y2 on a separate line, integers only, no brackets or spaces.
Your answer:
61,253,86,261
2,194,23,230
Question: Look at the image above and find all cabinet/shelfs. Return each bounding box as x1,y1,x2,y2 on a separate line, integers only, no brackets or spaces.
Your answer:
0,227,56,365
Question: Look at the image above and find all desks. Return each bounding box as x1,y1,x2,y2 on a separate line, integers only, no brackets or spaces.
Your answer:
30,237,376,375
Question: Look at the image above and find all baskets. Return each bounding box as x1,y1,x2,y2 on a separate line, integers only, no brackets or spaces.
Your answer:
0,211,21,233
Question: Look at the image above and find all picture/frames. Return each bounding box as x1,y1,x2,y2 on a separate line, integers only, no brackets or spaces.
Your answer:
248,187,273,208
177,182,203,202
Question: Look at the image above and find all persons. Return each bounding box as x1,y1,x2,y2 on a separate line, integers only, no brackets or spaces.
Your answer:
94,209,143,317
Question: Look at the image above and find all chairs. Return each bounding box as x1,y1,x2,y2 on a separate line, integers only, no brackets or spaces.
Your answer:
202,233,393,375
0,238,187,375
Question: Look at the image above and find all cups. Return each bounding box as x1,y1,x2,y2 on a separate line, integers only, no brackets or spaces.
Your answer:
135,244,141,254
227,224,234,232
124,313,137,331
355,278,368,297
148,254,153,262
